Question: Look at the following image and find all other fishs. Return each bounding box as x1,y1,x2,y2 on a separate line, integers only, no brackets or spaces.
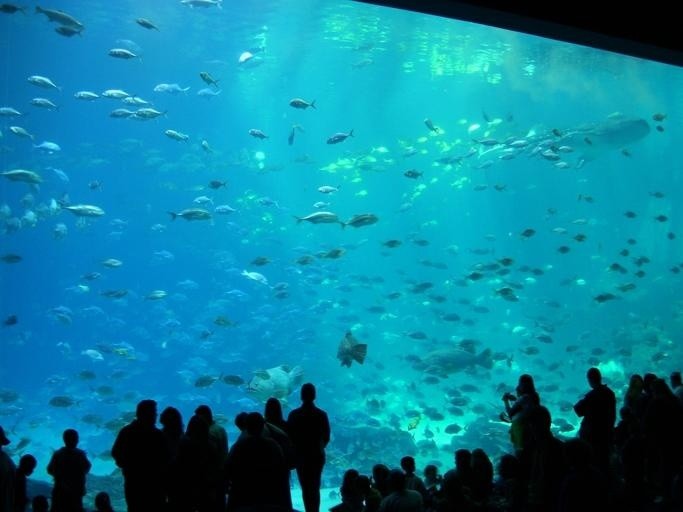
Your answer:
0,0,682,500
